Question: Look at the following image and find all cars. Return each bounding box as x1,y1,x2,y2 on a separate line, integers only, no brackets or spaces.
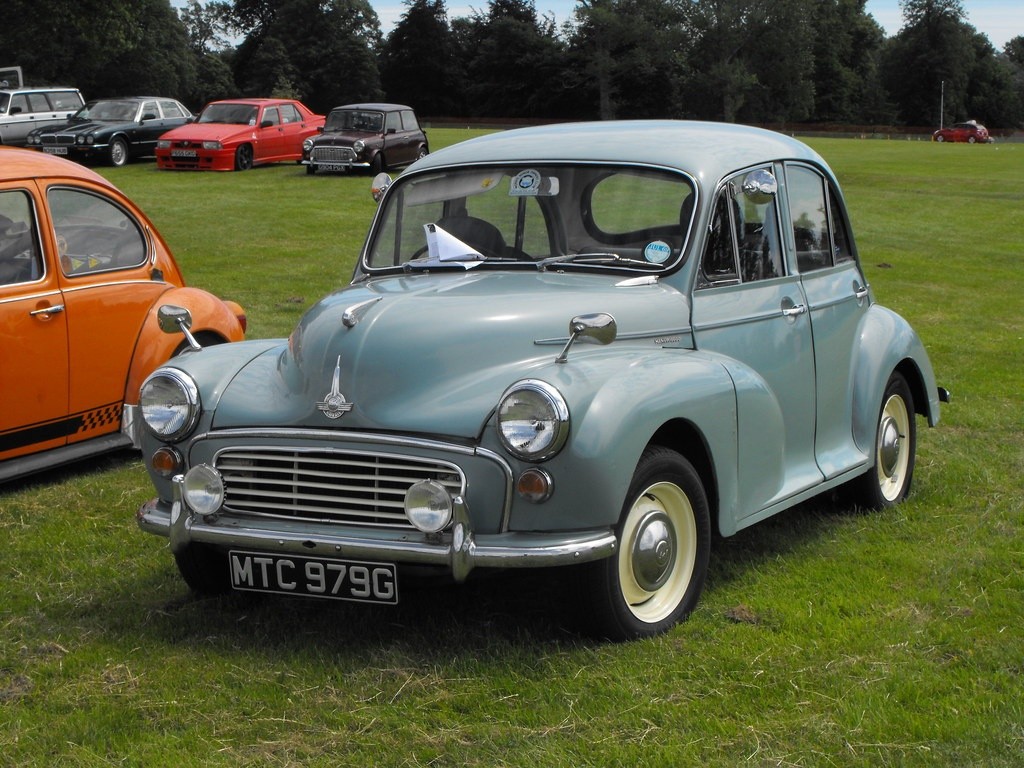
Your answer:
301,102,429,178
24,95,196,168
154,99,326,173
0,142,248,482
132,119,955,643
933,121,990,144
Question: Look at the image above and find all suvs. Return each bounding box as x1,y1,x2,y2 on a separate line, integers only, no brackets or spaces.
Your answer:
0,86,91,149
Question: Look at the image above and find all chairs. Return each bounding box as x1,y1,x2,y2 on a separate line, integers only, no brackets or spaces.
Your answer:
0,214,32,286
679,191,765,287
435,217,536,262
